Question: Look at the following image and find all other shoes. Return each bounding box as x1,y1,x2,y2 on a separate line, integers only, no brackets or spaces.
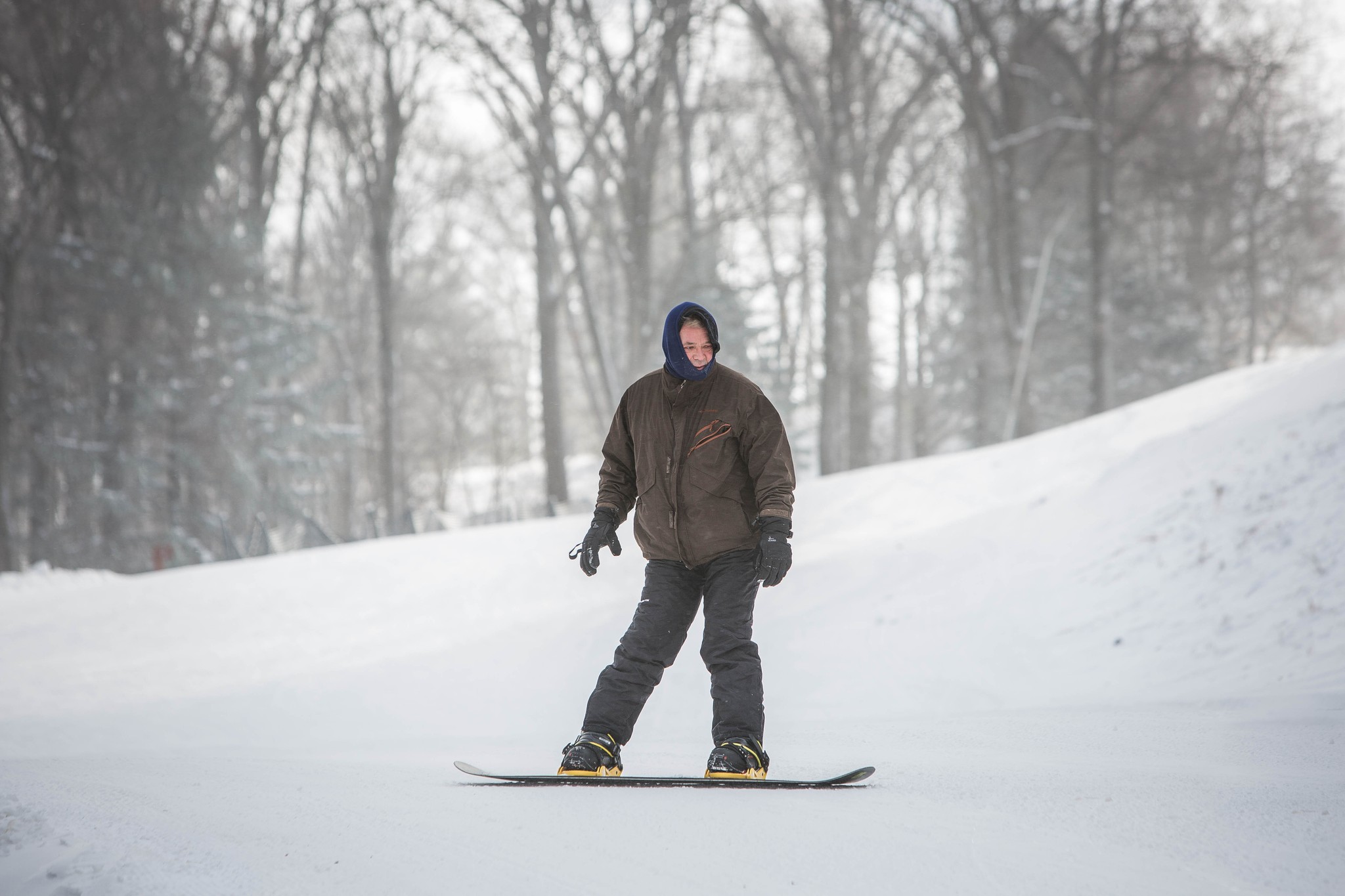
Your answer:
557,731,626,778
703,738,768,780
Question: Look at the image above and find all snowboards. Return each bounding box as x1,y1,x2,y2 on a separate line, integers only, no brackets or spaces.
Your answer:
452,757,875,787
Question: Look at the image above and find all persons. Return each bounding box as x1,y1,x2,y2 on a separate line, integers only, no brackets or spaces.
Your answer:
557,301,796,772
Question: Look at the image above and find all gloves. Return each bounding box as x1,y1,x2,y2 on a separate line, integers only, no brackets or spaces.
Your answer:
569,506,622,577
753,515,794,588
